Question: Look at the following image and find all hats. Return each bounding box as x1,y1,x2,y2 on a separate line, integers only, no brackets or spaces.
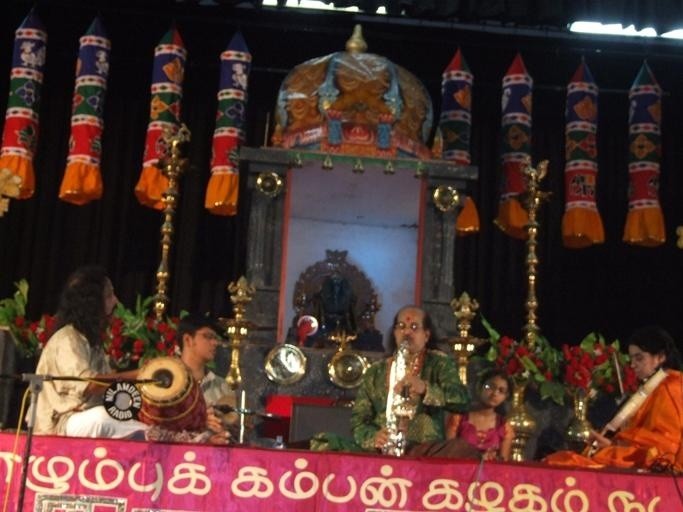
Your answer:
178,309,216,330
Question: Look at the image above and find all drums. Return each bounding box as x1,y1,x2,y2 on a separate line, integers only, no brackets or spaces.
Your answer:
136,357,207,431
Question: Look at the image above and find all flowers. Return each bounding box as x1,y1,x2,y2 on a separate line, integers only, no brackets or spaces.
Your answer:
479,311,639,394
0,275,190,370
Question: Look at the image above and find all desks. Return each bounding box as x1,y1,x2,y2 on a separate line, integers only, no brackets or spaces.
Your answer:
0,429,683,512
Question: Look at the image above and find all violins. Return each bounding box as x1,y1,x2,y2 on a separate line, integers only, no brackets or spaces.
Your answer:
583,369,667,458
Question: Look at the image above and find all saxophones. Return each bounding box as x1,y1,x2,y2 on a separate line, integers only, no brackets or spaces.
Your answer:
385,338,418,456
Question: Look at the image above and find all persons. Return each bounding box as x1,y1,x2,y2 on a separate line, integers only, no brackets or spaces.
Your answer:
143,311,236,449
445,368,516,466
307,303,483,465
541,322,683,476
20,263,147,441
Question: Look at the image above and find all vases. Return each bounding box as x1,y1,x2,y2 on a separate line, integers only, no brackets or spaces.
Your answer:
564,391,594,453
504,383,538,464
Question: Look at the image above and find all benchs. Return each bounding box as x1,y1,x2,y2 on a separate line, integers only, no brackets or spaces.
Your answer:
287,403,351,449
